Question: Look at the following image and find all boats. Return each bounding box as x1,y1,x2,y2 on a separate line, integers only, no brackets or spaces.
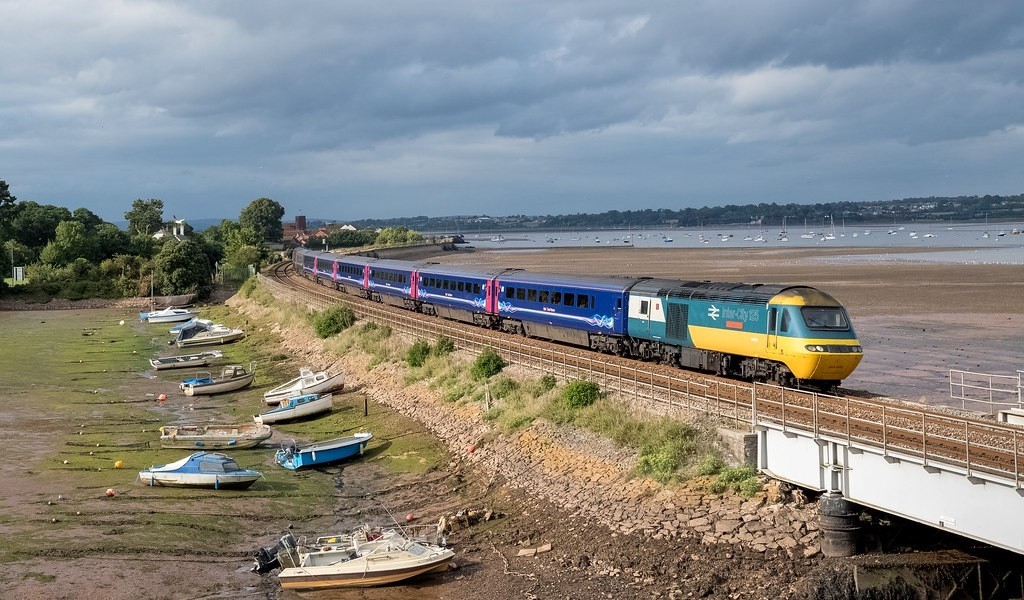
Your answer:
263,367,345,405
139,451,263,490
159,425,273,451
273,433,374,470
490,233,506,243
138,306,202,324
148,350,222,371
249,518,456,591
178,363,257,396
169,316,243,350
253,394,333,425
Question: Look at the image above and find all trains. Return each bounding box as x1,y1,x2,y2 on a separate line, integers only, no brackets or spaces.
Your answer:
292,247,865,391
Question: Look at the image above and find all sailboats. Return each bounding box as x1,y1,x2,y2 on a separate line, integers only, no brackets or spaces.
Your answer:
540,211,1022,245
